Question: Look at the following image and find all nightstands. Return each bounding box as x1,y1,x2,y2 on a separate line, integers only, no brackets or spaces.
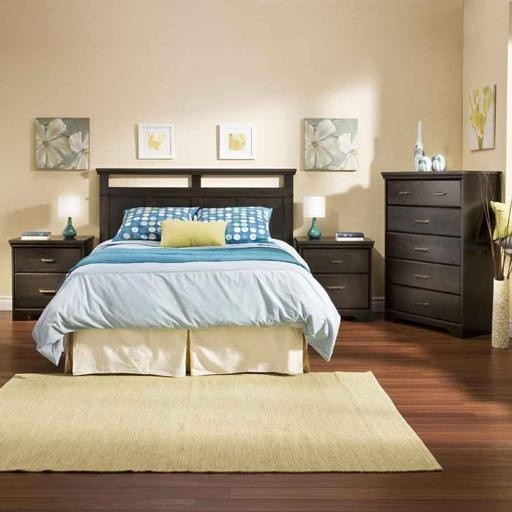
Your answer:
294,236,375,322
9,235,96,322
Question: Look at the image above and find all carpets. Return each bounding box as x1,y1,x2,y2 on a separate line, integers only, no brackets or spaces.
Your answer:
0,371,445,472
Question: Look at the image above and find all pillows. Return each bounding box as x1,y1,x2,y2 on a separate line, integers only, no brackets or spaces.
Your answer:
160,220,227,248
489,201,512,239
106,207,199,245
195,207,276,246
493,236,512,247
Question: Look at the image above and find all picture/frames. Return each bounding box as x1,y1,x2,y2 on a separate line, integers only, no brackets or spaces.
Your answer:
218,124,255,160
470,84,496,152
137,123,175,160
304,118,358,171
34,118,90,171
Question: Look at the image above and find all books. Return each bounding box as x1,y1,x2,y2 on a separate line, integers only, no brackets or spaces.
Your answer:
335,237,364,241
21,230,52,236
336,232,364,238
21,235,51,240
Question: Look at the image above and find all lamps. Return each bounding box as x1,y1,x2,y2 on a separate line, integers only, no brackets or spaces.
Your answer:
303,196,326,239
57,195,82,238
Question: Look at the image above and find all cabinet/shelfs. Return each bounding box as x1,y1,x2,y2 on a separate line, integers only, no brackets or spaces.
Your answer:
382,171,501,337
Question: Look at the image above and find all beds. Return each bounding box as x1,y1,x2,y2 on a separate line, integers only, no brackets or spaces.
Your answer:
31,168,341,379
